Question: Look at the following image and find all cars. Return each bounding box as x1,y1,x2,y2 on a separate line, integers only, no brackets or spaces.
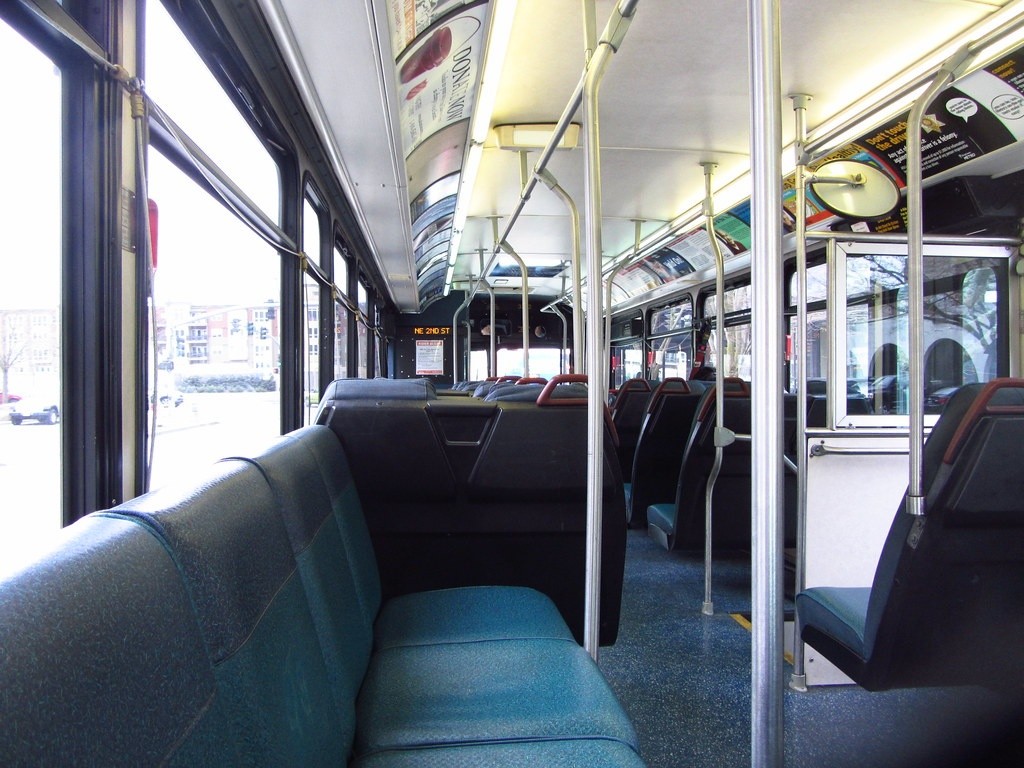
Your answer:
8,382,61,423
848,375,909,410
926,387,961,411
158,370,184,409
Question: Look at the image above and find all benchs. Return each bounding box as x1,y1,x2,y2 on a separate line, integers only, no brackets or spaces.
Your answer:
0,374,1024,768
